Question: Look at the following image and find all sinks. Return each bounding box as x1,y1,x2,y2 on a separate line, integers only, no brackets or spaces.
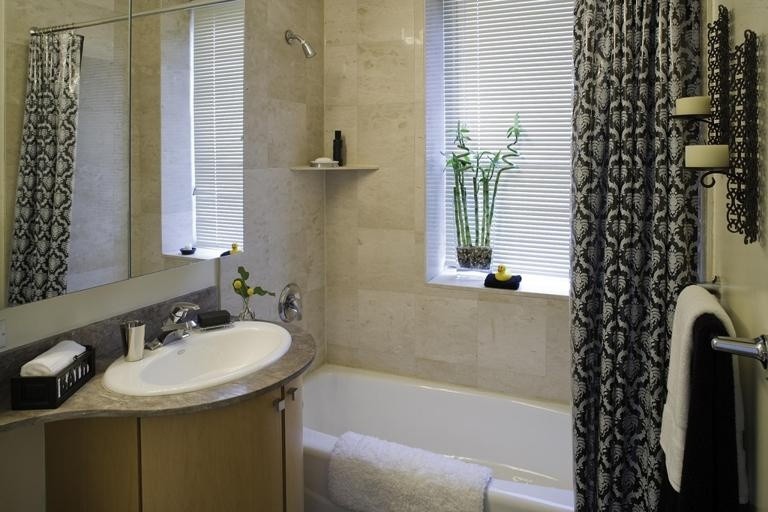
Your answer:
101,319,292,397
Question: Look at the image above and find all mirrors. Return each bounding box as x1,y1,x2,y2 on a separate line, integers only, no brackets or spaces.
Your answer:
0,1,246,309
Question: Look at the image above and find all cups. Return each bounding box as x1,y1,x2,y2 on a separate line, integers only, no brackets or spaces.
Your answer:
120,320,145,362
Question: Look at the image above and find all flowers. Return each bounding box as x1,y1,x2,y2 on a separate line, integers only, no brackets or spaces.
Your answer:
232,265,277,306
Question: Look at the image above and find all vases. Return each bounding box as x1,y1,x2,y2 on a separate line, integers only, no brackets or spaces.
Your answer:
238,304,256,321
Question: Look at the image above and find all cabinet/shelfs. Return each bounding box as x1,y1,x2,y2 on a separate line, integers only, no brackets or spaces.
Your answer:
0,375,317,511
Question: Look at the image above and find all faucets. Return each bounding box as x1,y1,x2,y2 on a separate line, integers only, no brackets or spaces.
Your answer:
145,302,200,350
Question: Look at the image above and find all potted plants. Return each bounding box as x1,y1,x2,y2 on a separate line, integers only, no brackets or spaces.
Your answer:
439,111,521,270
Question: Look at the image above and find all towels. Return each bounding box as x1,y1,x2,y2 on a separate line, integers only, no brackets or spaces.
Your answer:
653,314,740,512
658,284,750,505
327,431,493,512
20,339,90,398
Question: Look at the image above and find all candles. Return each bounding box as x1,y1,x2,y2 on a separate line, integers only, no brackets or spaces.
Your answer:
676,91,711,115
685,144,729,167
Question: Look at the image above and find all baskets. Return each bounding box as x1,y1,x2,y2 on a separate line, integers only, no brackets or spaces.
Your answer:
11,344,95,410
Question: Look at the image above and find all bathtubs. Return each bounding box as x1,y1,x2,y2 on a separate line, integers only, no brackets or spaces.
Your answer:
303,363,577,512
